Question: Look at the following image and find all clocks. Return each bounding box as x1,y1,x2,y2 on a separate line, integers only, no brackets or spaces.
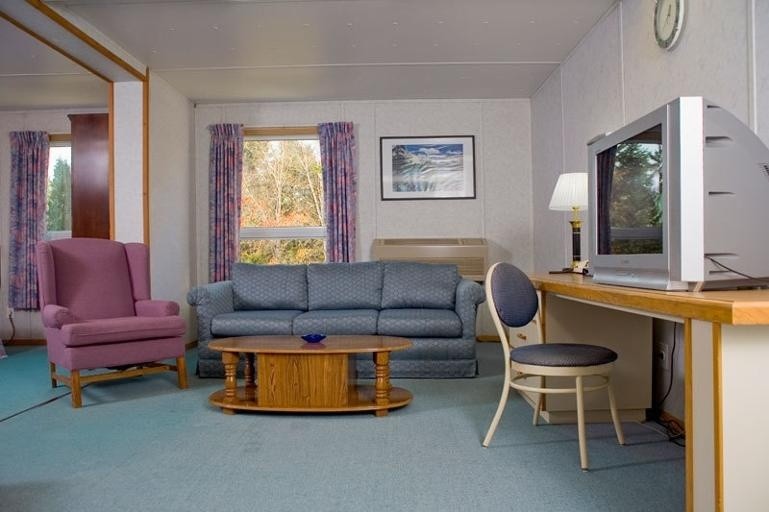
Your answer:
652,0,685,51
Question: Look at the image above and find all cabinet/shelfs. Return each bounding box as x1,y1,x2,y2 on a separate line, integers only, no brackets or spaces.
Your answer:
67,113,109,239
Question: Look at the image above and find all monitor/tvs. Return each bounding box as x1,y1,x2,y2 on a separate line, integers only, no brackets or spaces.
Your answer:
586,94,769,291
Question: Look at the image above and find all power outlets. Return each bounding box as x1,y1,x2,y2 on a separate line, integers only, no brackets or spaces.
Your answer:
7,307,14,319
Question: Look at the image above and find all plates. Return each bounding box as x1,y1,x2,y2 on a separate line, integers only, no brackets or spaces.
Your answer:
300,333,327,344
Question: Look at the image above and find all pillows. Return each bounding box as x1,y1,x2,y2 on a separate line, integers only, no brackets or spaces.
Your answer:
228,263,458,309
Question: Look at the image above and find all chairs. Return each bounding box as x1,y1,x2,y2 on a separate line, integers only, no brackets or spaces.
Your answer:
36,238,186,407
482,262,624,473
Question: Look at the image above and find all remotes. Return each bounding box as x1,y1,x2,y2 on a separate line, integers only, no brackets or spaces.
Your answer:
585,131,610,146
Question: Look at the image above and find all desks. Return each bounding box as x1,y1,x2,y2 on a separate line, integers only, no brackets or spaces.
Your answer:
507,273,769,512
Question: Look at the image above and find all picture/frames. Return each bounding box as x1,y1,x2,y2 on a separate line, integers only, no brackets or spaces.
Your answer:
380,136,476,200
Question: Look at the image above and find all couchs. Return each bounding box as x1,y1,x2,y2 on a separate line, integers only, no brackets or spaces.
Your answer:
187,281,486,379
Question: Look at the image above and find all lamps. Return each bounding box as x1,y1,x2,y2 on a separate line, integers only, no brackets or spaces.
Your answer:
548,173,588,274
